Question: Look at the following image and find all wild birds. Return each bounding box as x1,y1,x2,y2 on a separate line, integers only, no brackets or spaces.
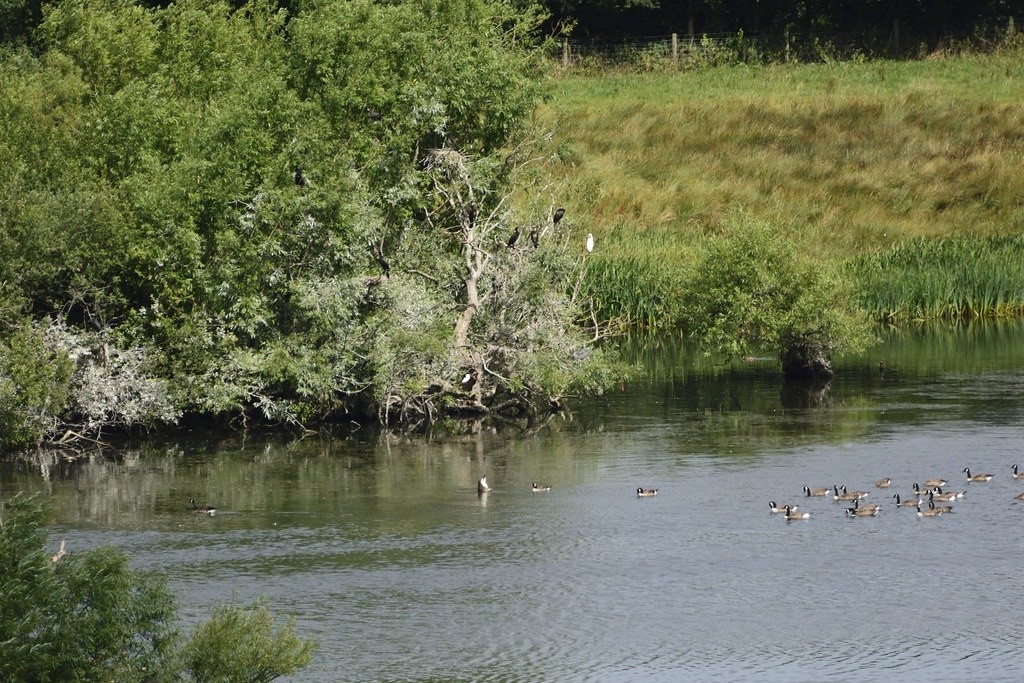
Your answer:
531,481,553,493
477,474,496,492
879,359,885,372
366,208,596,279
188,498,220,517
455,366,478,384
636,487,659,497
768,463,1024,521
46,539,67,563
